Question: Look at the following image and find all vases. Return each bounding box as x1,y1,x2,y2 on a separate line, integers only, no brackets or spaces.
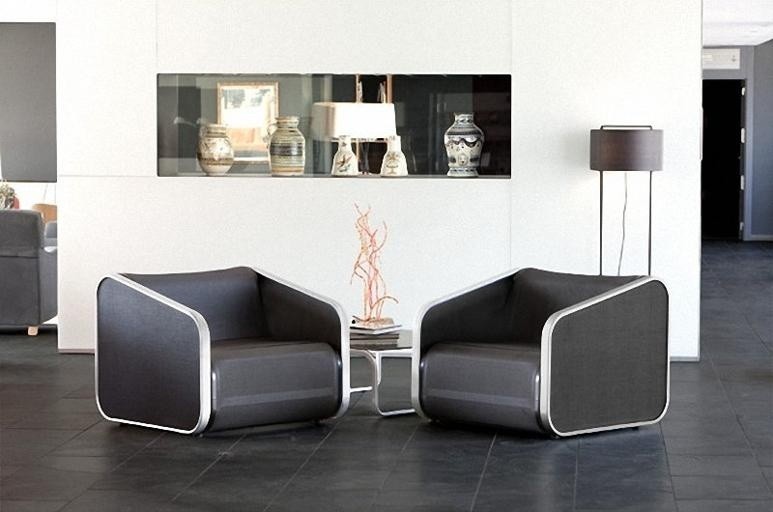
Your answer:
196,111,486,179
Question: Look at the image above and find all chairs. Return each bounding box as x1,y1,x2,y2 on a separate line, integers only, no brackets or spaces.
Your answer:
0,209,56,336
94,265,350,438
409,266,669,441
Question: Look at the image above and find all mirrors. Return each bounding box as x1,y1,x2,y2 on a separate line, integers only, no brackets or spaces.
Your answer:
216,81,279,165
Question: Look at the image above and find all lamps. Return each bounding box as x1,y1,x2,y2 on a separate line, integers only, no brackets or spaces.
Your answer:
589,125,661,276
